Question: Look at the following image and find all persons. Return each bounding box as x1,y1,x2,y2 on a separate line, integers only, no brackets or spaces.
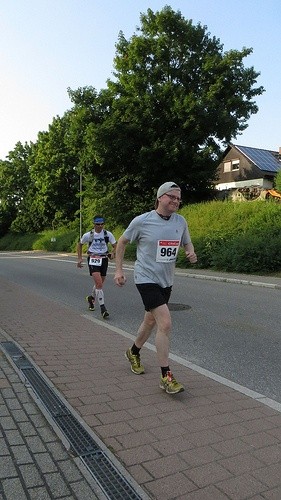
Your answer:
114,181,197,393
77,214,117,317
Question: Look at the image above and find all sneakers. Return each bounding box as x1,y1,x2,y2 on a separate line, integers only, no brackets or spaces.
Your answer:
124,348,144,375
159,371,184,394
102,310,109,318
86,295,95,310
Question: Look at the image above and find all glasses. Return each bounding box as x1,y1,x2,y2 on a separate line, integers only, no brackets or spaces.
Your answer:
95,223,104,225
165,194,180,202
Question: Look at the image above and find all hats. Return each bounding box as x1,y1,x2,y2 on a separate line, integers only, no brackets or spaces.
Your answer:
156,181,181,206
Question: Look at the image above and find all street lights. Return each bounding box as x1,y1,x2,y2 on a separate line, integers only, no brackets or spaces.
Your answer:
73,167,82,241
